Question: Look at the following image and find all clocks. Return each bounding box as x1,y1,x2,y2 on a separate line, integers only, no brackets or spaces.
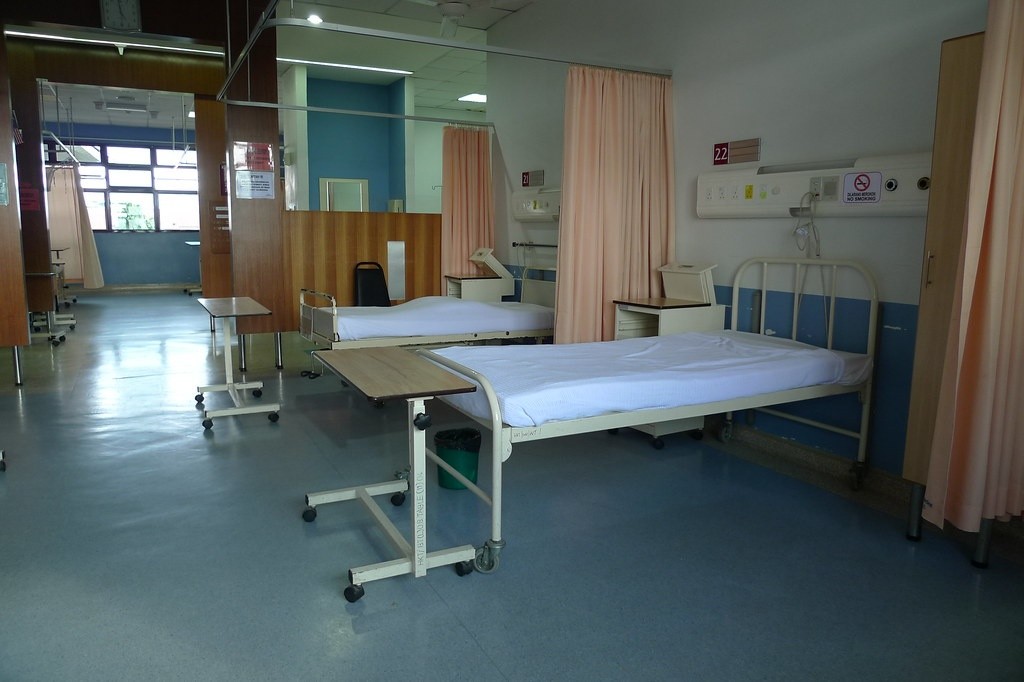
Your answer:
99,0,143,33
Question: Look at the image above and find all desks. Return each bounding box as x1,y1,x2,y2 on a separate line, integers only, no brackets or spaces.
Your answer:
294,343,478,604
184,241,202,295
196,295,283,430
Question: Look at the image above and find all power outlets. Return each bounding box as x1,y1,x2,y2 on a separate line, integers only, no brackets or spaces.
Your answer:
705,183,754,201
809,176,822,201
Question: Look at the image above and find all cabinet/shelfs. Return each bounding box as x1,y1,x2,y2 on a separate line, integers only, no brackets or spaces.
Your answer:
445,273,502,302
612,296,725,444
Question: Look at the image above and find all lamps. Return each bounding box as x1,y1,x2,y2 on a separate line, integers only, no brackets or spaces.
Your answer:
437,2,472,19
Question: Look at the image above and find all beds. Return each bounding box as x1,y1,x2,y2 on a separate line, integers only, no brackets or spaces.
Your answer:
416,256,884,575
296,263,558,406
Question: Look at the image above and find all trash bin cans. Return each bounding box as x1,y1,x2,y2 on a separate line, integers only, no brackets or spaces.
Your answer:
434,427,481,490
